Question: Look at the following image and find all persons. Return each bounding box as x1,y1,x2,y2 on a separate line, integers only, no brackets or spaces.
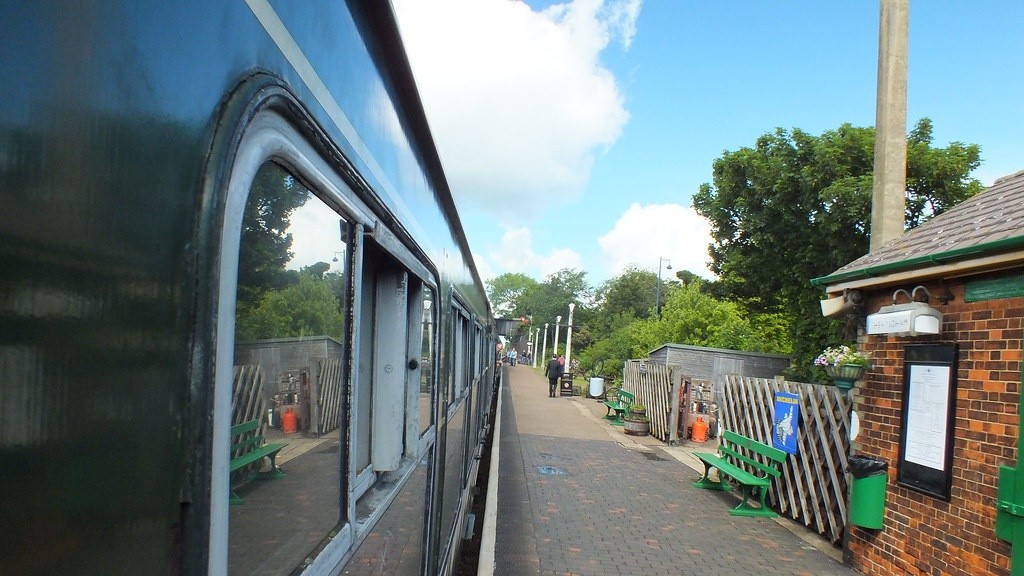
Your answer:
544,353,561,397
507,347,533,366
557,354,565,374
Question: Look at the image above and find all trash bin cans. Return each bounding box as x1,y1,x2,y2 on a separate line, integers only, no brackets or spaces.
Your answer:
560,373,573,396
848,454,888,529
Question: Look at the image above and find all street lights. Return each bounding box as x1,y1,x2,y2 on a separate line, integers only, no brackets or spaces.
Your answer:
655,257,672,315
526,303,576,377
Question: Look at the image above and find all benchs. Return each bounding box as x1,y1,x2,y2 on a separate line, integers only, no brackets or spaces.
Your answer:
229,419,290,504
690,428,788,518
605,389,634,425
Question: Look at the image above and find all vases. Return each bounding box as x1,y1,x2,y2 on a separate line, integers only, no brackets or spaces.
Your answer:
824,364,864,389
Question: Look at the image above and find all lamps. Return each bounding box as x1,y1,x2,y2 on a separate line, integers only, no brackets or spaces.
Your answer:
865,285,946,337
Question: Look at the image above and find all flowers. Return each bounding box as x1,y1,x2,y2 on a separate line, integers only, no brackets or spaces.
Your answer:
812,343,874,376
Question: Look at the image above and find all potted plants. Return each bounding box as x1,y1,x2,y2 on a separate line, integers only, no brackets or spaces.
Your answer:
623,403,649,436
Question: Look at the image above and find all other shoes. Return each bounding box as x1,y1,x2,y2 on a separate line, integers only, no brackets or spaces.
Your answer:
548,395,552,397
552,393,556,397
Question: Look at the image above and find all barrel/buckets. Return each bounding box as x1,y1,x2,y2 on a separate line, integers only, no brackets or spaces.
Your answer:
283,408,298,434
590,378,604,396
691,416,708,442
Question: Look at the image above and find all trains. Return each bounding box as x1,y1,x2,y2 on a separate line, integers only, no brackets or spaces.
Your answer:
1,0,496,574
